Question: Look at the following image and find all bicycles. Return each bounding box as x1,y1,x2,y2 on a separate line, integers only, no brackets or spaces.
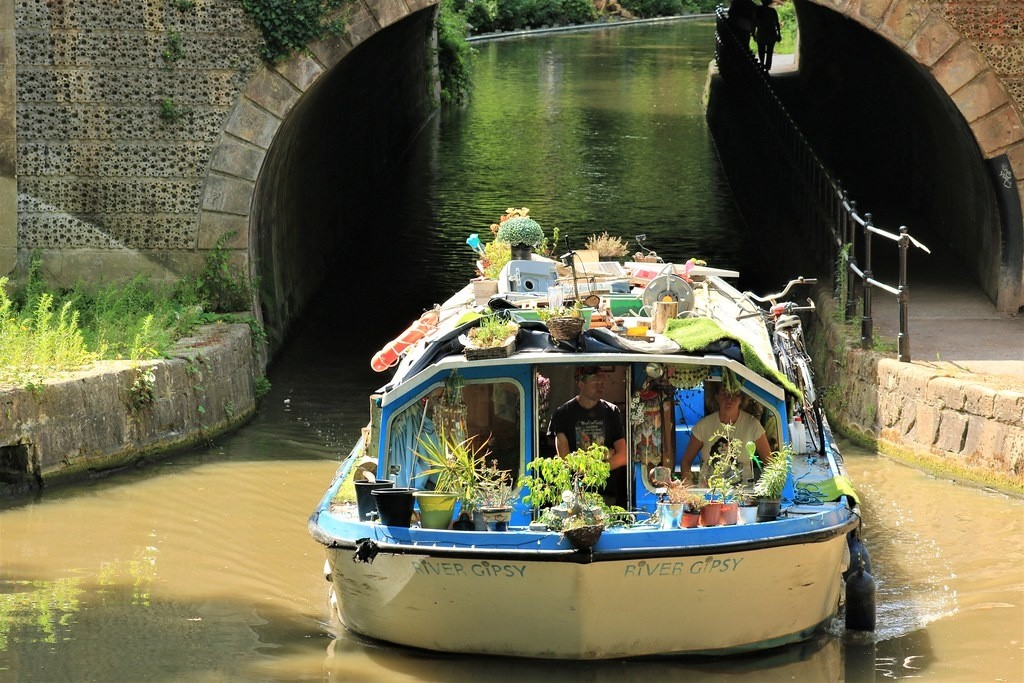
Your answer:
736,274,825,455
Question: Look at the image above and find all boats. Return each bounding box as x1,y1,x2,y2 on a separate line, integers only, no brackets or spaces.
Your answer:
309,206,877,662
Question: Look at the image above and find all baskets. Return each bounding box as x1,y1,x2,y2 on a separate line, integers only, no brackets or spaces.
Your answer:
565,524,604,550
546,315,585,340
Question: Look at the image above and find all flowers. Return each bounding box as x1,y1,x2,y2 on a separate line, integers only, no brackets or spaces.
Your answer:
465,207,560,283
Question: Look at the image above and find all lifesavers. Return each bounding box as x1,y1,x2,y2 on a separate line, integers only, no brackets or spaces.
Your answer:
369,309,439,373
641,275,695,319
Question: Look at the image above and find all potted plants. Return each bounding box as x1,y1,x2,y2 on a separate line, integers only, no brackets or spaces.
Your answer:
408,435,515,532
454,307,521,361
649,420,797,528
496,216,544,260
535,300,585,341
518,443,610,551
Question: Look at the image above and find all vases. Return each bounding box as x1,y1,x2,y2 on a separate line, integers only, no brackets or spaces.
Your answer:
371,487,420,529
353,480,394,524
471,278,500,307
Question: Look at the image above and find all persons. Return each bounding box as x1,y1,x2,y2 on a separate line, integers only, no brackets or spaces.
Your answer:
546,367,628,508
682,386,773,491
751,0,781,69
